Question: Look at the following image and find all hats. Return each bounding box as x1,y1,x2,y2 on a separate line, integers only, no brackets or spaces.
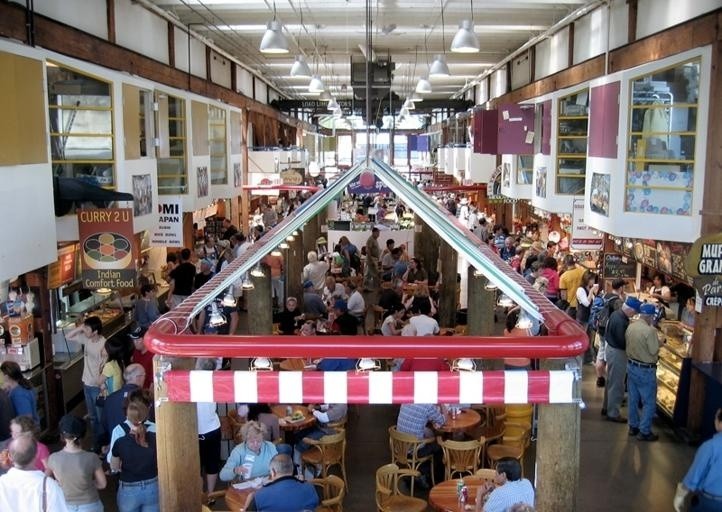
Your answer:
625,296,656,314
612,279,628,288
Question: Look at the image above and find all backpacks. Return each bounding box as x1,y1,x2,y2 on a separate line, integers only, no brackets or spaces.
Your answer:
589,293,618,334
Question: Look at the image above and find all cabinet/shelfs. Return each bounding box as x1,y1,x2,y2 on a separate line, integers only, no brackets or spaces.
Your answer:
52,292,126,365
594,318,692,426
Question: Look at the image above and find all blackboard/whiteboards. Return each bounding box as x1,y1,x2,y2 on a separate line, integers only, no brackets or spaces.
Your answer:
603,253,637,280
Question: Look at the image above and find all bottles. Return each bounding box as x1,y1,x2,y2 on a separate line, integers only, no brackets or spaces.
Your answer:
457,480,465,496
461,488,468,503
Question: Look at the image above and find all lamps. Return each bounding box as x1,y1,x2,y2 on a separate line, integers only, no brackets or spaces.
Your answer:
395,0,480,129
260,0,352,131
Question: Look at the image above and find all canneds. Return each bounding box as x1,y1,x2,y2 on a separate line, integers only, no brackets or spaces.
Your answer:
460,486,468,502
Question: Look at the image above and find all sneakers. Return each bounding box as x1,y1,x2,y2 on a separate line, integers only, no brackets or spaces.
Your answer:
597,377,605,387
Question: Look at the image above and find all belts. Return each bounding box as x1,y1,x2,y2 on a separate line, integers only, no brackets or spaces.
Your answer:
627,360,655,368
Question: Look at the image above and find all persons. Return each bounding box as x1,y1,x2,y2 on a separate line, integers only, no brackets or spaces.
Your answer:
1,166,722,511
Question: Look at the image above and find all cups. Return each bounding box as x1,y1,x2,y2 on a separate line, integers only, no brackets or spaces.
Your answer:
285,406,292,416
241,463,253,479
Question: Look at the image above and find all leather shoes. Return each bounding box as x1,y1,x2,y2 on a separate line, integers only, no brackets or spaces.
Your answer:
601,408,628,423
628,427,659,441
